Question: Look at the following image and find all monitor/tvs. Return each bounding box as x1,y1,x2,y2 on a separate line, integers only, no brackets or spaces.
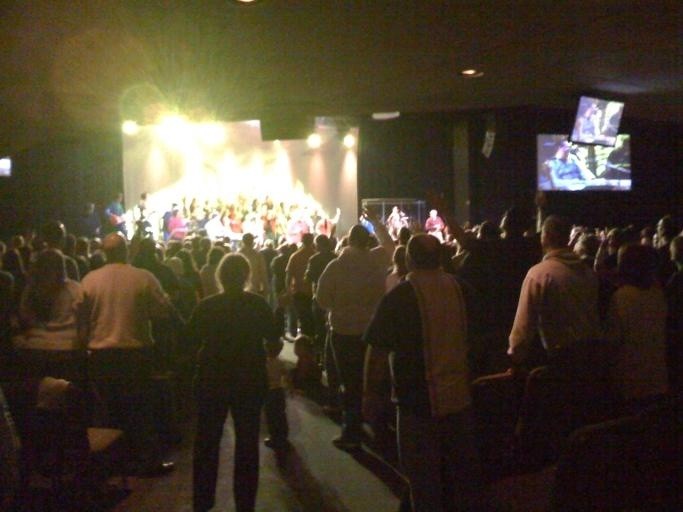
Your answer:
534,132,634,194
568,93,626,147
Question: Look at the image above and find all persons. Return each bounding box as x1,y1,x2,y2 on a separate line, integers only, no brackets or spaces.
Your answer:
271,193,682,510
2,191,288,510
599,135,632,180
547,139,610,190
572,102,608,144
607,107,623,130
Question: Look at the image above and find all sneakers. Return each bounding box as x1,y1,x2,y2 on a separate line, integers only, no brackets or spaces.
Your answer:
330,435,362,452
147,460,177,477
261,435,292,449
